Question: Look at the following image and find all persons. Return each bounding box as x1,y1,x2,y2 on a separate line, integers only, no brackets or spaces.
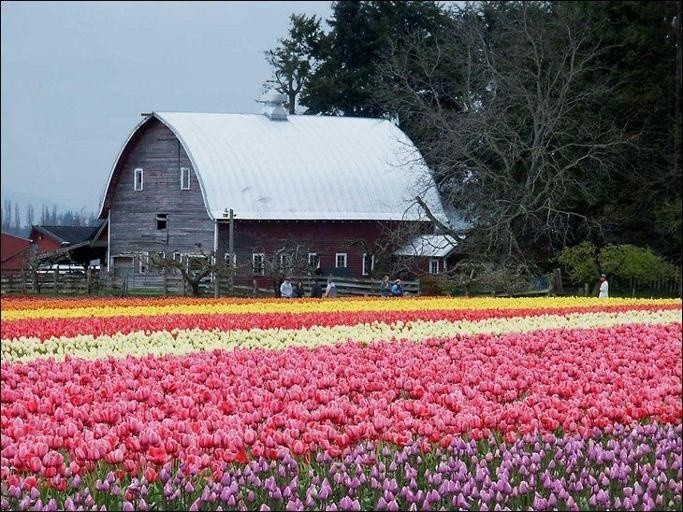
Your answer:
598,274,609,297
294,281,304,297
325,280,337,297
309,280,322,297
392,277,403,296
280,274,293,300
380,275,399,297
